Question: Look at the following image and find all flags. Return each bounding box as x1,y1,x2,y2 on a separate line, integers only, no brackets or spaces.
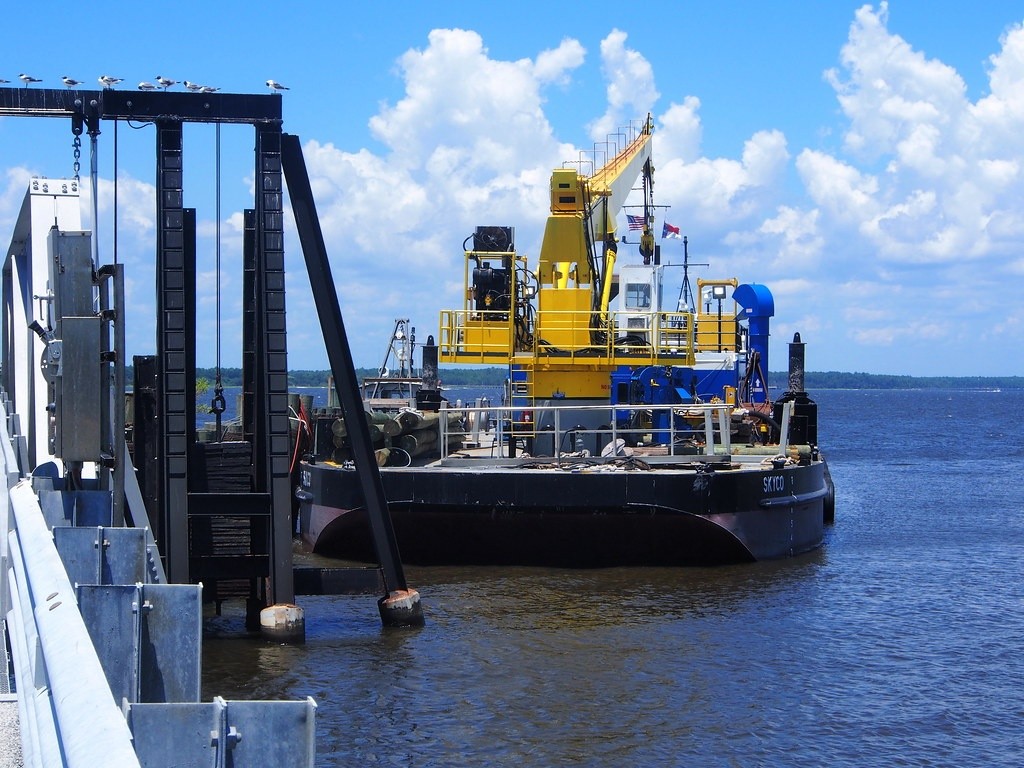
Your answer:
661,222,681,240
627,215,644,230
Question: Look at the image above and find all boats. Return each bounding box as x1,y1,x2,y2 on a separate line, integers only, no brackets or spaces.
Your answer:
438,386,446,391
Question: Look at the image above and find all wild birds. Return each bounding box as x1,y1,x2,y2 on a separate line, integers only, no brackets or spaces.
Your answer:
0,73,43,88
61,76,84,90
266,80,290,94
183,80,221,93
97,75,125,91
138,75,181,92
